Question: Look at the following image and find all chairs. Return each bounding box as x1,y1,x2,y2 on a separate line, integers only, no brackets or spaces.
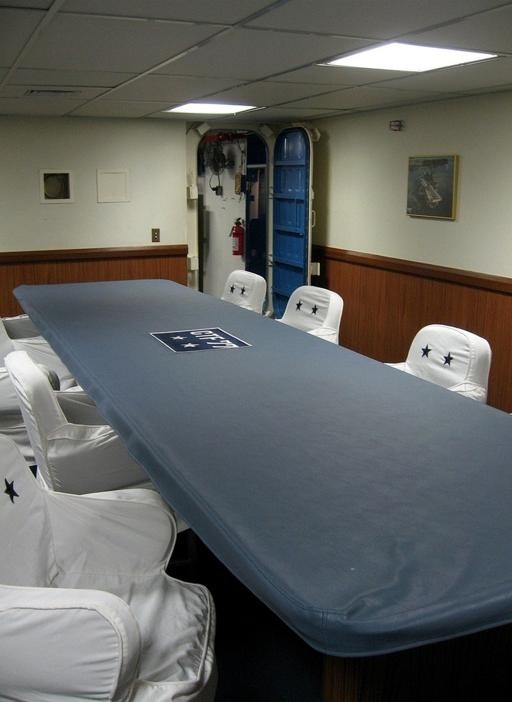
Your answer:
0,314,83,392
276,284,344,346
386,323,492,403
0,433,218,701
222,270,266,314
5,351,191,533
0,365,109,468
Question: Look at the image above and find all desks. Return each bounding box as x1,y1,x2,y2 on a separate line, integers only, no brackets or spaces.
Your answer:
14,278,511,702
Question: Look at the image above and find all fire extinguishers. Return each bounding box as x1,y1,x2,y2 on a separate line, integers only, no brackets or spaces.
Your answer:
229,217,244,256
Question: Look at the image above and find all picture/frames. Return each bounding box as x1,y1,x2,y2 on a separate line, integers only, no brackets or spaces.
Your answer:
406,152,458,220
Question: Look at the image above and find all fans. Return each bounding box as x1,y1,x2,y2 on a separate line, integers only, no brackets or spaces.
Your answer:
208,151,235,175
